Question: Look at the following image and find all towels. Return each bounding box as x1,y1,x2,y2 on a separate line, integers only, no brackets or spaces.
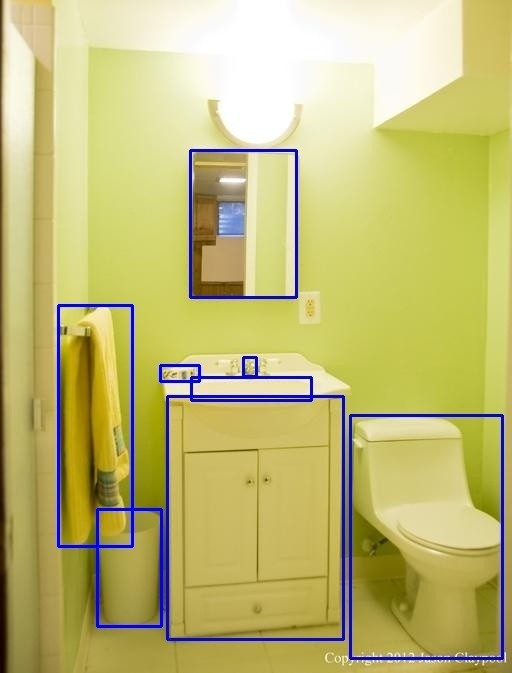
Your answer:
59,306,132,546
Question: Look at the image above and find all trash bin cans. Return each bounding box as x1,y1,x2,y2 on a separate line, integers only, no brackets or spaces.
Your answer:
98,509,162,627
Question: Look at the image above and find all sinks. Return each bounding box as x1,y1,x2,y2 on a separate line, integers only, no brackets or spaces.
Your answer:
191,378,312,401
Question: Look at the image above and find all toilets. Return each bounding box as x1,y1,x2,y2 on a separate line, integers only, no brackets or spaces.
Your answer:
350,415,503,659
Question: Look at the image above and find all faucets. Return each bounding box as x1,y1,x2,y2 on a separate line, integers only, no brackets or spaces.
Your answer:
244,357,257,377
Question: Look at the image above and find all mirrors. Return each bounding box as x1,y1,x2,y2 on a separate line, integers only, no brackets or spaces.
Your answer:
190,150,297,297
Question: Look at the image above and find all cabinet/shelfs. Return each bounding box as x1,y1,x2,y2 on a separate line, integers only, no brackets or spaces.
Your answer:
167,396,343,639
